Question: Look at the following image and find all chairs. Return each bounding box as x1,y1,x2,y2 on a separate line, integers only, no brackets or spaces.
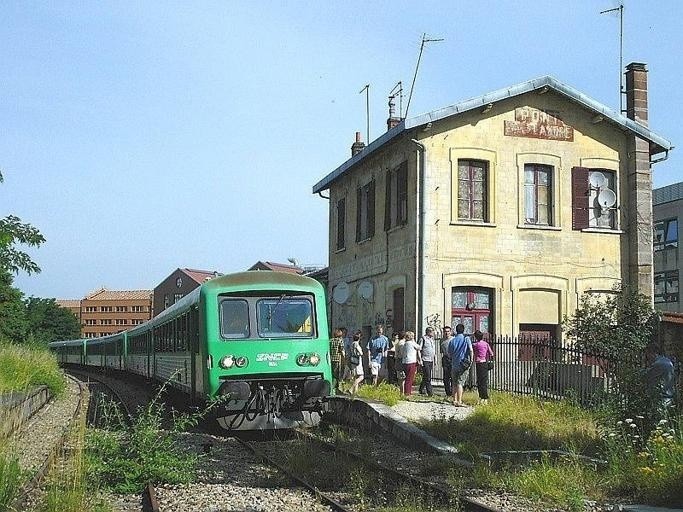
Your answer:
452,401,467,407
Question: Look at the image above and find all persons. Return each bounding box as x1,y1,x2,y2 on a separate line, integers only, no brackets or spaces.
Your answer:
393,330,405,395
365,324,390,387
386,331,402,385
416,327,438,398
447,323,474,408
328,328,351,396
400,331,422,398
471,330,495,406
640,344,675,408
439,325,456,398
344,333,365,398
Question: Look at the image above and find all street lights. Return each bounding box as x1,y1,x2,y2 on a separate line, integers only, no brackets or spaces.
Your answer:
462,358,472,370
488,363,494,370
350,356,359,364
398,371,407,380
